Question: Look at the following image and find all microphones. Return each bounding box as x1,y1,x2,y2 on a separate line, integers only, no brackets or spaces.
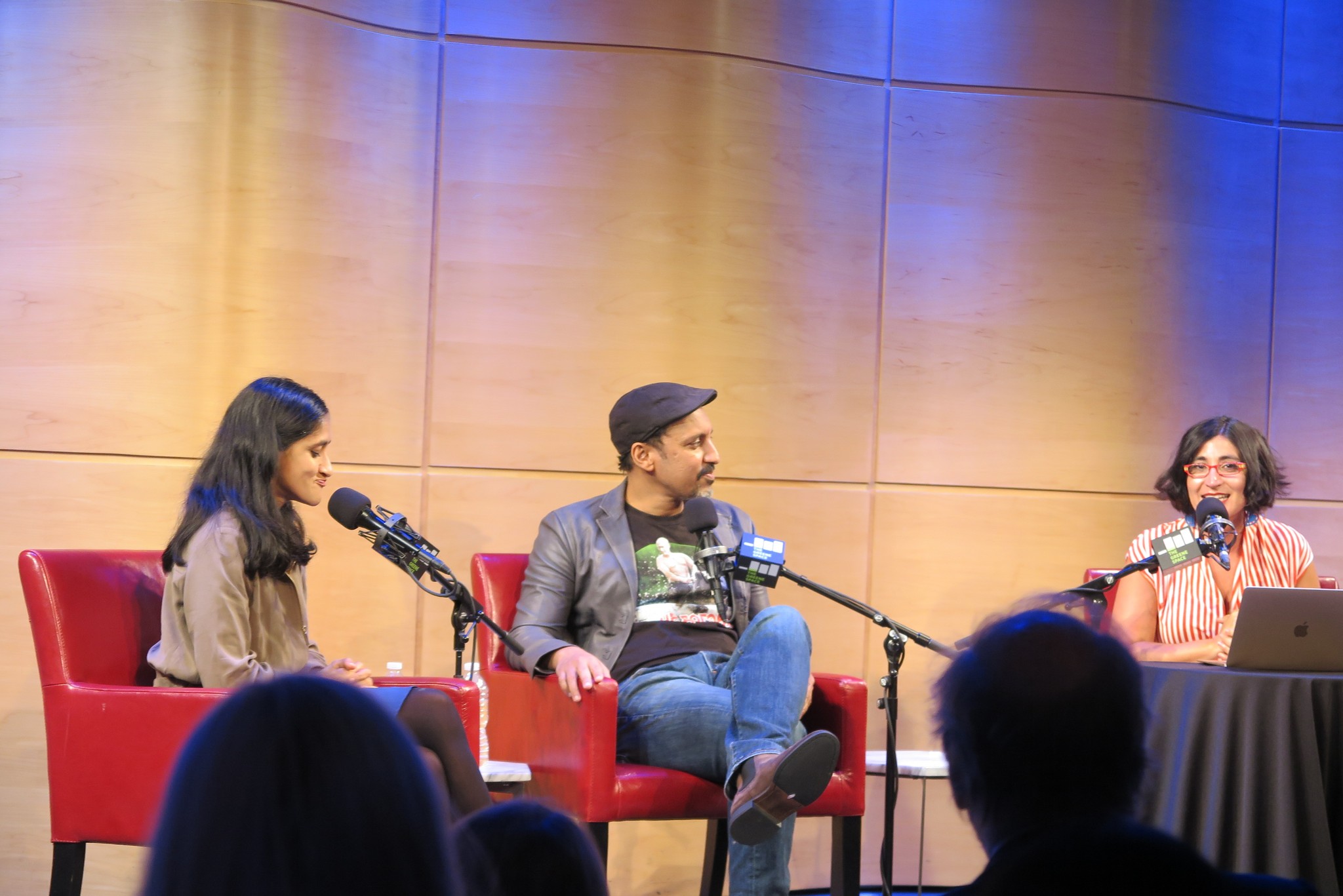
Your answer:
1196,497,1231,570
684,496,730,619
328,487,453,575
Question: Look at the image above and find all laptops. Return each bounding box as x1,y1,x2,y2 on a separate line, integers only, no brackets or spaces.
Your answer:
1197,586,1343,672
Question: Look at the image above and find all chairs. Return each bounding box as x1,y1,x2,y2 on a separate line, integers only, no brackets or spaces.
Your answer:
468,546,869,896
1078,563,1339,636
18,544,484,896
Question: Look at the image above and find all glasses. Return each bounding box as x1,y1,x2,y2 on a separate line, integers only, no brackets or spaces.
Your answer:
1184,461,1247,478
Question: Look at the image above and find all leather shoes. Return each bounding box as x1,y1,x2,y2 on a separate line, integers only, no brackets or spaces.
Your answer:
729,730,840,847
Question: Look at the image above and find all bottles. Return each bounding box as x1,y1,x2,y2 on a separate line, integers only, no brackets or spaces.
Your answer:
463,662,490,769
387,661,402,677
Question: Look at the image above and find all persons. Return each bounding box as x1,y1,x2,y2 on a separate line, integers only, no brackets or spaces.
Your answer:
150,674,615,896
924,609,1317,896
144,376,496,826
507,381,843,896
1112,416,1322,671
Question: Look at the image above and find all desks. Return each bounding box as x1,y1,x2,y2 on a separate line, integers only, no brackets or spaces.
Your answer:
864,745,970,896
1127,654,1343,896
478,758,535,803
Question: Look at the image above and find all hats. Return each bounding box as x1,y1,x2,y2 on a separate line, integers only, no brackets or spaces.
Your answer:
608,382,718,456
